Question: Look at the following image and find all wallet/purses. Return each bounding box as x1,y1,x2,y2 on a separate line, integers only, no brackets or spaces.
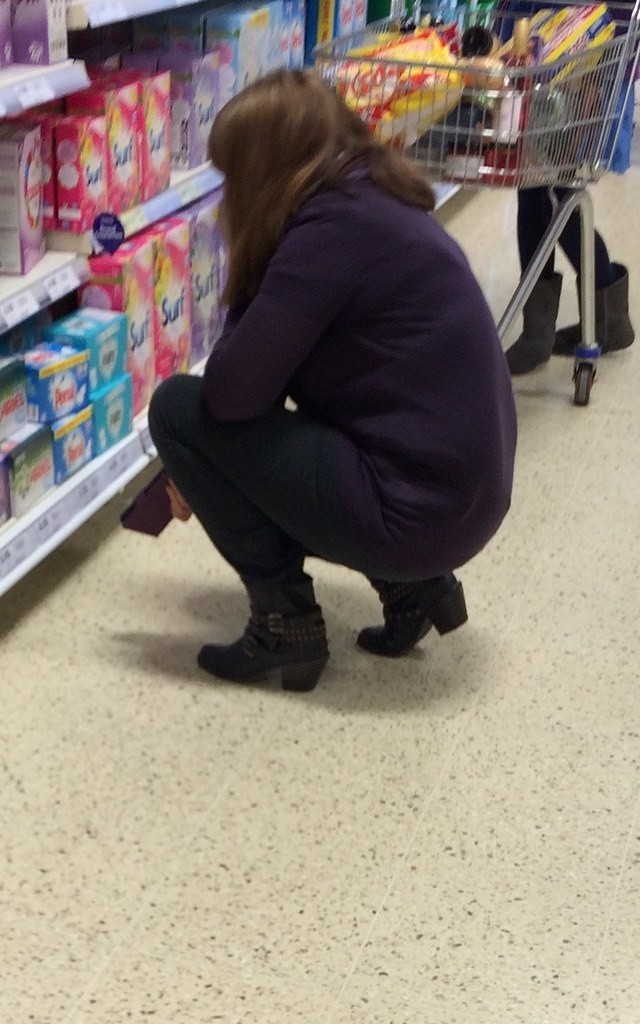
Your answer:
120,467,175,537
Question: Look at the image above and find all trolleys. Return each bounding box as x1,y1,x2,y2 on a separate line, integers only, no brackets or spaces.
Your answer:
315,1,640,412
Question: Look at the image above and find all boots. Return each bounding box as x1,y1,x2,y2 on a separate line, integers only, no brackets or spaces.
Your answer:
553,262,636,355
506,271,563,375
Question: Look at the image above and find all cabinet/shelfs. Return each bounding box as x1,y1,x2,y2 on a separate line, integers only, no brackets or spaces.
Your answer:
65,0,476,467
0,56,151,598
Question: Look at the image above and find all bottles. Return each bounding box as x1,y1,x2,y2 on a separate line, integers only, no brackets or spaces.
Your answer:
482,17,533,184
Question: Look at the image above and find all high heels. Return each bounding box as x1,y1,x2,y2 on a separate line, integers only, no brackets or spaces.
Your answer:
197,573,331,691
357,573,469,658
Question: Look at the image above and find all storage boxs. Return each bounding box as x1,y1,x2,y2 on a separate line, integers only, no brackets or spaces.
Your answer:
0,0,619,512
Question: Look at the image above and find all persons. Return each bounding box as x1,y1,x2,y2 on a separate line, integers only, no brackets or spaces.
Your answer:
492,1,639,375
147,70,518,693
